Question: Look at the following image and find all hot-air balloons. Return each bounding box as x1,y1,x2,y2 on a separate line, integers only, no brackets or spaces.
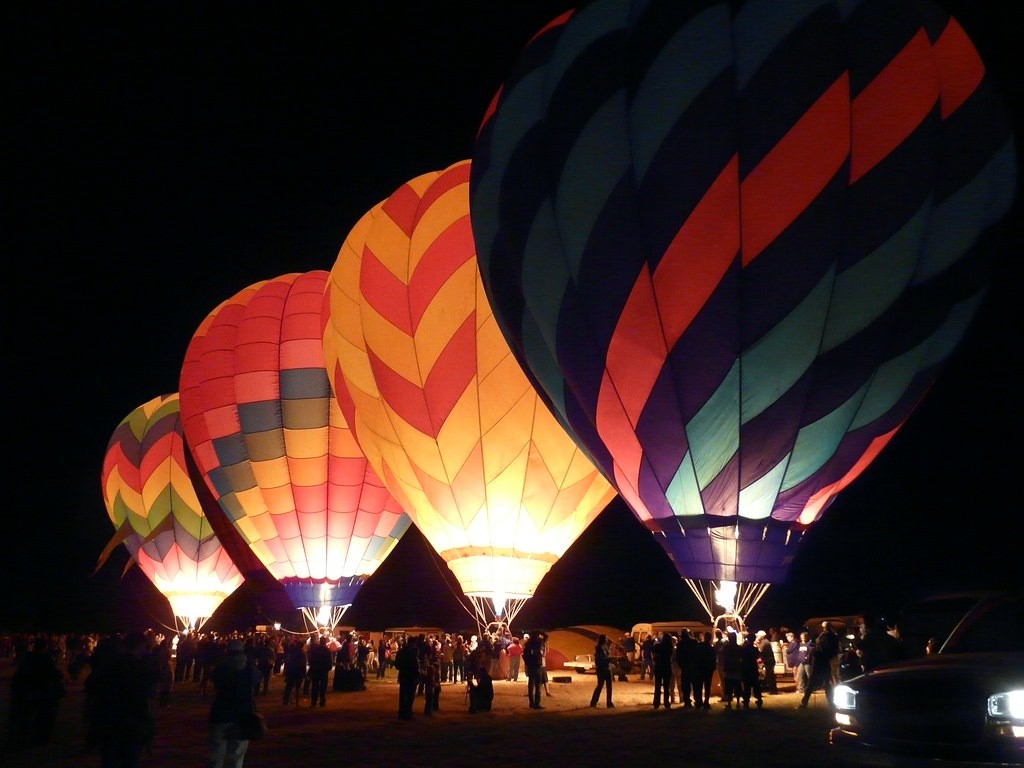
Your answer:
102,391,247,664
468,0,1017,698
177,269,411,692
321,159,619,680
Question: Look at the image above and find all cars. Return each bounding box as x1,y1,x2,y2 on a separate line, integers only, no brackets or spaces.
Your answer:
802,589,982,667
829,595,1024,767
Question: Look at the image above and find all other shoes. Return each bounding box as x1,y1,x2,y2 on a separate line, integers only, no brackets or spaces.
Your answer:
530,702,535,708
607,703,614,707
666,706,671,712
534,704,545,709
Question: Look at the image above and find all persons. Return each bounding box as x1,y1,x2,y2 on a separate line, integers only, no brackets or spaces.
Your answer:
0,617,985,768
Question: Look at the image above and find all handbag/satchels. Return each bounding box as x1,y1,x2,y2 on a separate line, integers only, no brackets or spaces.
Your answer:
244,711,267,739
